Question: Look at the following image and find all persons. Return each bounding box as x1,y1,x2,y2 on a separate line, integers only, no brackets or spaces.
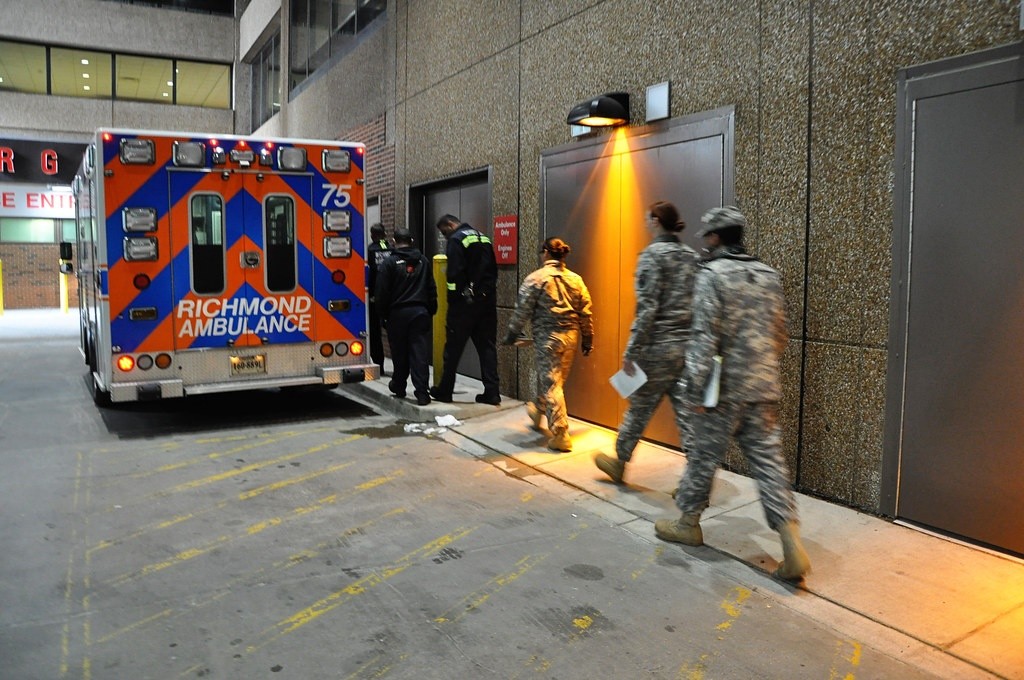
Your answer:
368,224,395,376
596,202,711,508
653,205,811,579
501,237,594,451
429,214,502,405
374,228,438,406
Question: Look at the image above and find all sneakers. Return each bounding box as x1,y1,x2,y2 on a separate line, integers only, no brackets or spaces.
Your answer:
380,363,385,376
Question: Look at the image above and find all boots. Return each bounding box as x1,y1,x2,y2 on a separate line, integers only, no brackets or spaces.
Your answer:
672,488,710,508
772,521,811,580
656,513,703,548
548,426,573,452
526,401,541,425
595,453,626,483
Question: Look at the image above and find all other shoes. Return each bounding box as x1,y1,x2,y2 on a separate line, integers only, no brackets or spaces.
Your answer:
430,386,453,403
414,389,431,406
388,380,407,398
475,392,501,405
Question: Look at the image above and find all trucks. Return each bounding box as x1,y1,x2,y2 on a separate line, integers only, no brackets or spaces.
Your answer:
55,127,383,409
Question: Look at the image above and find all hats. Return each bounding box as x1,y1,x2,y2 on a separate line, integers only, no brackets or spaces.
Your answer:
694,205,746,238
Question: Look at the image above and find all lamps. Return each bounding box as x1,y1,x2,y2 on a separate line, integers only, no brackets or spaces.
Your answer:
567,91,632,129
